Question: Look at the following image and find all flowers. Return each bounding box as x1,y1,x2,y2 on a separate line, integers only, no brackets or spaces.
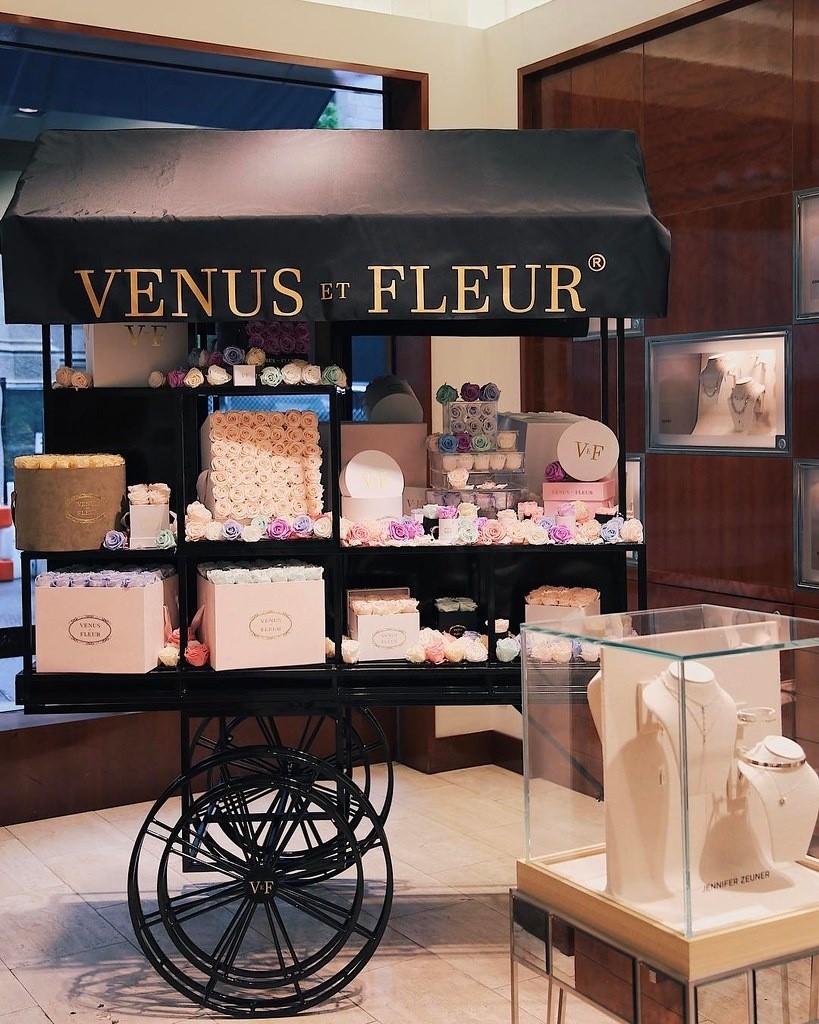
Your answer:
52,366,94,391
525,583,600,607
155,529,179,550
185,410,332,542
128,483,171,506
405,627,489,663
36,563,175,588
103,530,129,551
485,619,611,663
149,322,348,388
159,626,210,668
339,382,643,546
14,452,125,469
435,596,479,613
325,636,361,665
350,594,421,614
198,558,325,583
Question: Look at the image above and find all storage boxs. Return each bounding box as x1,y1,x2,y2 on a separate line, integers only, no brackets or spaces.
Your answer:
498,413,591,496
253,353,308,386
443,403,497,434
200,409,323,525
426,487,530,514
543,478,616,502
345,588,419,660
494,430,520,452
543,498,614,518
197,573,326,673
526,600,601,624
429,451,525,475
35,575,180,673
429,469,523,492
14,464,126,551
339,420,428,494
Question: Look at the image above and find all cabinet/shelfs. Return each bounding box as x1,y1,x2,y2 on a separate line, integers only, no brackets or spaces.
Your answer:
14,319,651,714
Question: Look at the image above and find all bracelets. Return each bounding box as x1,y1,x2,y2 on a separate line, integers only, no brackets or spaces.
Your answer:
737,707,777,721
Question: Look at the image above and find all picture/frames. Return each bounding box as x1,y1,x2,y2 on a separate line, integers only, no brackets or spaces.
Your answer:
644,325,791,457
792,186,819,325
792,457,819,593
573,315,646,343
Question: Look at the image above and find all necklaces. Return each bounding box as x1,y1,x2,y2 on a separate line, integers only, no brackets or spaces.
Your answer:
740,753,807,804
702,368,723,397
660,674,724,742
730,388,752,413
